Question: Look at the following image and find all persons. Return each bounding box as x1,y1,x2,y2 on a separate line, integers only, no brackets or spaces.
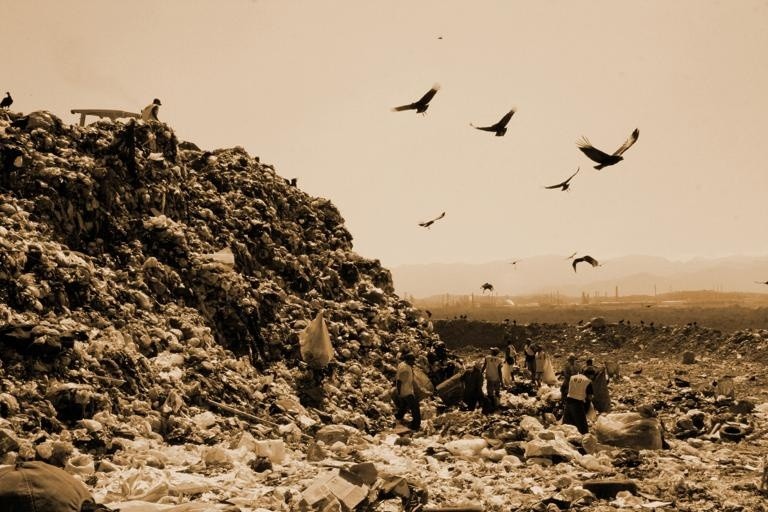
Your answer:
482,346,504,404
393,350,421,432
502,337,519,382
534,343,550,389
141,98,163,122
581,358,606,415
561,371,594,437
554,352,577,408
523,337,541,386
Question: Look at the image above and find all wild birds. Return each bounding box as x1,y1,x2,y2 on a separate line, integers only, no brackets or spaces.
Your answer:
503,319,517,327
578,319,584,326
543,127,640,194
0,92,14,111
561,248,599,272
417,210,446,229
633,366,756,389
687,321,697,327
389,82,438,118
480,282,493,293
468,107,522,138
644,304,654,307
453,314,469,319
508,259,521,265
618,317,656,327
